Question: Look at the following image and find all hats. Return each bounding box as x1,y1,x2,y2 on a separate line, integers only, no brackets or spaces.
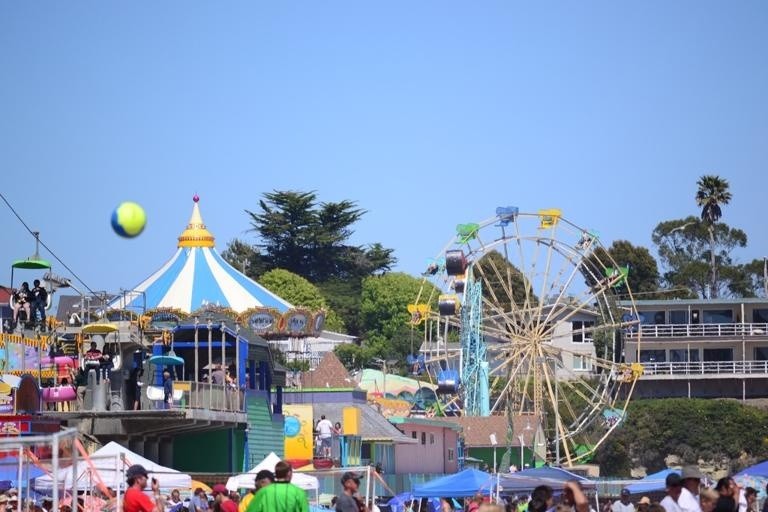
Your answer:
636,496,650,505
126,466,152,478
341,472,364,484
256,471,276,479
666,473,685,487
682,465,704,480
209,484,229,495
745,486,759,497
621,489,629,495
0,494,18,505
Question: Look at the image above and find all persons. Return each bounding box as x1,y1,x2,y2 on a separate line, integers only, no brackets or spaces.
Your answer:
316,415,341,457
58,341,237,412
30,280,47,321
333,422,344,435
10,282,33,323
